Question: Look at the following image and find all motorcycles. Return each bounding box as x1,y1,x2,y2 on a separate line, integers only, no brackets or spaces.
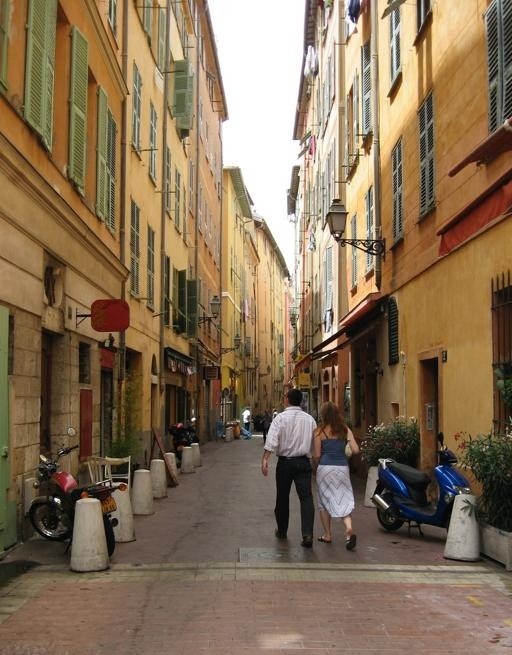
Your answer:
368,429,476,540
167,414,202,462
25,426,127,561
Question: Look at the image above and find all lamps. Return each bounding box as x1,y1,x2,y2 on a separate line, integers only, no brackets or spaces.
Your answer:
198,295,222,324
221,333,241,355
325,198,386,263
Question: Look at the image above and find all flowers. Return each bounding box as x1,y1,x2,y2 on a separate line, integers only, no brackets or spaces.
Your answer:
358,415,418,470
448,415,512,531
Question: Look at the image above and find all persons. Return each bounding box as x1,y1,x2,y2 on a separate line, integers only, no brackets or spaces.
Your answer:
259,409,272,443
241,407,251,431
310,400,361,550
261,388,318,548
271,408,279,418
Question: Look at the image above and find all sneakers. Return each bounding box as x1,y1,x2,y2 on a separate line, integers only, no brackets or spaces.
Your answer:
274,528,287,539
301,536,313,548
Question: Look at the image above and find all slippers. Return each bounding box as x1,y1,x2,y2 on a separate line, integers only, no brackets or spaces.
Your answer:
345,534,356,550
318,536,331,543
